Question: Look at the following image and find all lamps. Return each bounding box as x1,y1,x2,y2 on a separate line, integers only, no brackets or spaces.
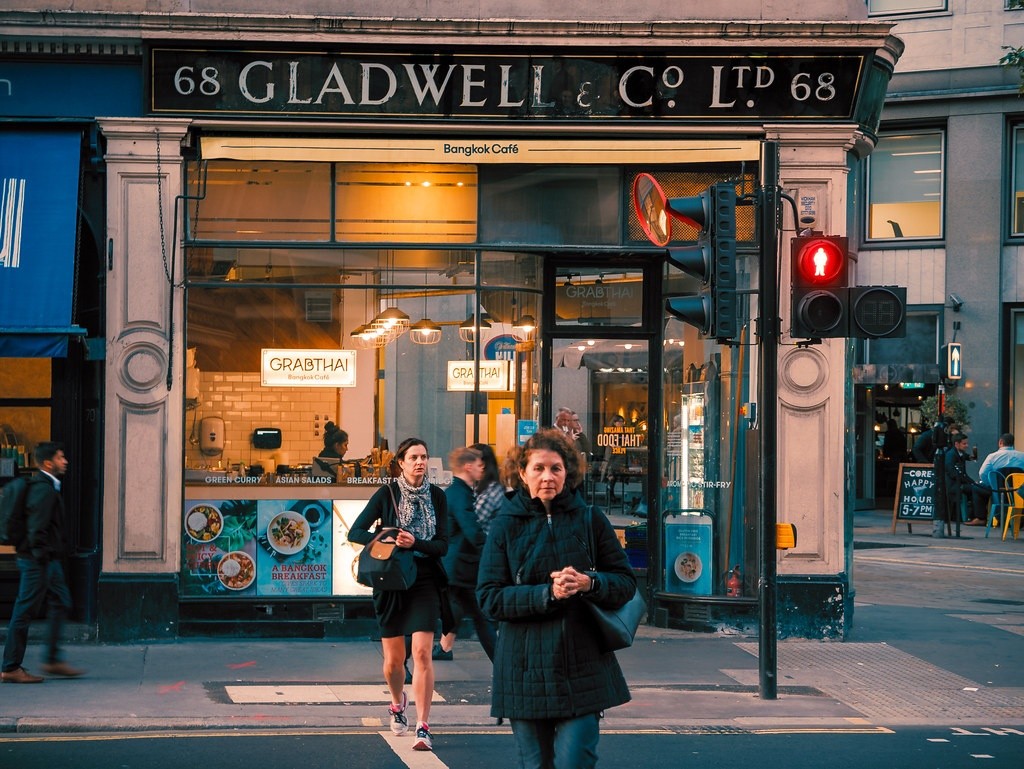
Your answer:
349,247,536,348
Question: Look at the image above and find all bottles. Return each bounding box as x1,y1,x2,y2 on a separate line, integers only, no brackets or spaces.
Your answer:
18,452,24,469
239,461,246,478
6,444,12,458
12,446,18,462
0,444,6,458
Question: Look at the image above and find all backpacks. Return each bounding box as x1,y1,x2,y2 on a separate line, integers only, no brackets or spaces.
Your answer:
0,476,57,546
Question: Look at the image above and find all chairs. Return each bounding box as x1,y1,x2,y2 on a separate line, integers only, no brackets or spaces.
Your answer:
580,448,646,518
984,467,1024,542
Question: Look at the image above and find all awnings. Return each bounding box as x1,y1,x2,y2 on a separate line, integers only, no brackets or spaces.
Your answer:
0,130,83,359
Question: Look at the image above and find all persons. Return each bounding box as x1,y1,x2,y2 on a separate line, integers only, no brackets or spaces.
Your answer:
948,425,959,435
912,417,955,464
348,438,448,750
318,421,348,462
965,433,1024,526
0,441,87,682
430,443,525,664
553,407,590,453
945,433,976,494
474,429,637,769
600,415,627,503
882,419,906,511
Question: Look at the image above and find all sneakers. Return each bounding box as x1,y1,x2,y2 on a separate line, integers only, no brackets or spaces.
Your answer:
412,723,434,751
388,691,408,736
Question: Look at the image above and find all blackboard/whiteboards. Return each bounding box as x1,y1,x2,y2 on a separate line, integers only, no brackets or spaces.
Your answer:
894,462,935,521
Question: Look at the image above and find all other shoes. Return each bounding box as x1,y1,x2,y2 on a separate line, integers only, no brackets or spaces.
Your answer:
39,664,82,676
0,666,44,683
403,666,413,684
432,644,453,660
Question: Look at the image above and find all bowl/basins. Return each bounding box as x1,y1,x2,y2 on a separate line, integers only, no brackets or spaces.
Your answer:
217,551,256,591
267,510,311,555
184,502,224,543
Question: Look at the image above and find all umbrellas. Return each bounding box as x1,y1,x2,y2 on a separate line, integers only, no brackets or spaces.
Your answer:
550,313,685,367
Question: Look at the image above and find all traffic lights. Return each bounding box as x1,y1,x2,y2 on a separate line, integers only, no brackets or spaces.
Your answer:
789,235,853,290
663,183,737,341
788,281,908,338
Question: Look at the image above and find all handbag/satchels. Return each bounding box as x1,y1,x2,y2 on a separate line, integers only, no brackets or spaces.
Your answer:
350,484,417,592
582,505,646,651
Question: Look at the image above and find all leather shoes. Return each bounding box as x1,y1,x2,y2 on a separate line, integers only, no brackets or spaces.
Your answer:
964,518,987,526
